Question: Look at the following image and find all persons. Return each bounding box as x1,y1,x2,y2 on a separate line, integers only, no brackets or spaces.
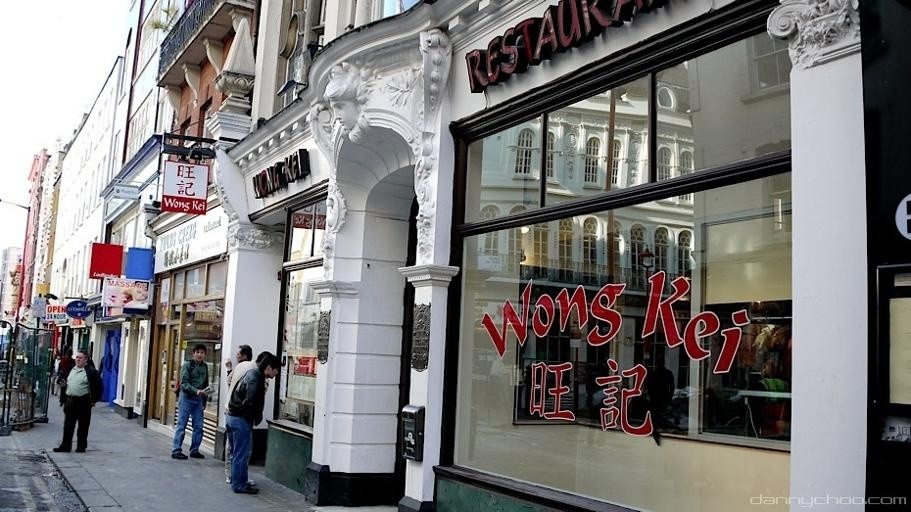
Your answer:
51,353,61,396
225,353,282,495
222,351,269,485
57,349,76,401
113,281,149,305
169,343,216,459
52,350,103,453
223,344,253,387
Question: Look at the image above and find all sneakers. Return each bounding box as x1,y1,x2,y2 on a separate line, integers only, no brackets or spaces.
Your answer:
189,452,205,459
172,452,189,459
54,446,86,452
226,475,260,494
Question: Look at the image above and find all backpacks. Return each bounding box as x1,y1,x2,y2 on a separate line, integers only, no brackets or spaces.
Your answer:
175,359,195,398
